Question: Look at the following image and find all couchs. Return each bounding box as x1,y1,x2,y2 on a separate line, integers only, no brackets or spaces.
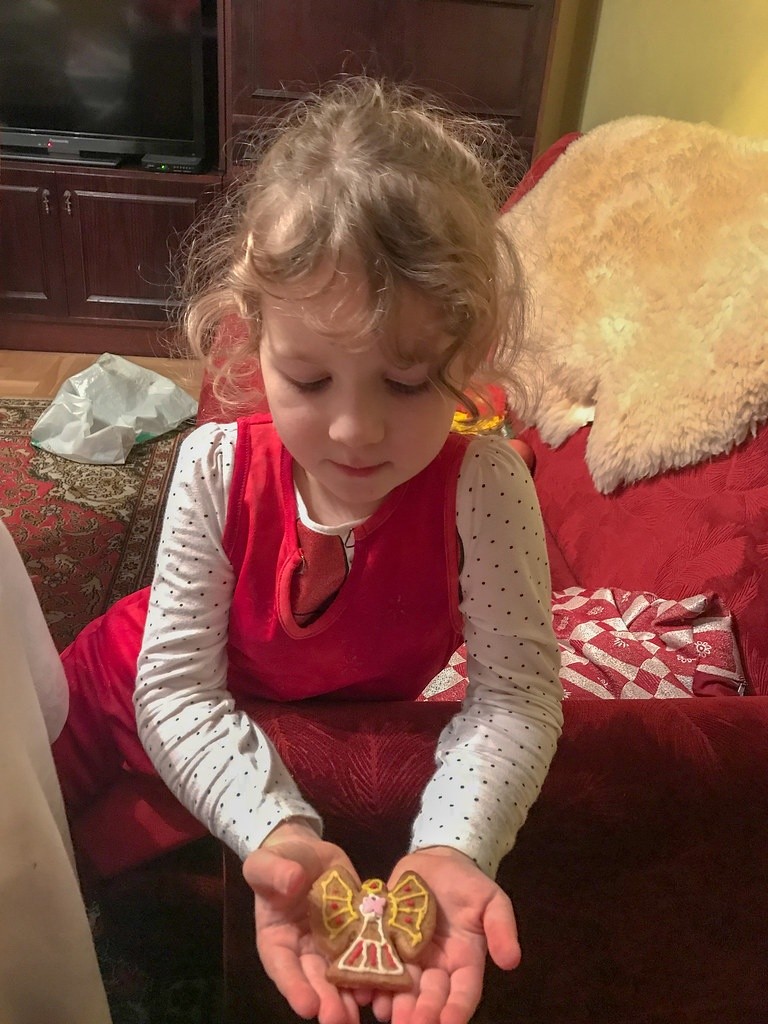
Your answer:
96,111,768,1024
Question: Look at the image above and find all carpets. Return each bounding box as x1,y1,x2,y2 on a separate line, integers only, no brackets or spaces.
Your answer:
0,396,225,1024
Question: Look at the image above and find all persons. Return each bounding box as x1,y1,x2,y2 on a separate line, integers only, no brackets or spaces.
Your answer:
52,74,566,1023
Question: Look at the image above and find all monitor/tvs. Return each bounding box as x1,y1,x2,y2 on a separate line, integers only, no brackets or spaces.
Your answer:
0,0,213,167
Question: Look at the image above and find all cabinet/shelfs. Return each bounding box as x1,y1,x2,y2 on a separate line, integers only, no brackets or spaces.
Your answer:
0,159,221,363
